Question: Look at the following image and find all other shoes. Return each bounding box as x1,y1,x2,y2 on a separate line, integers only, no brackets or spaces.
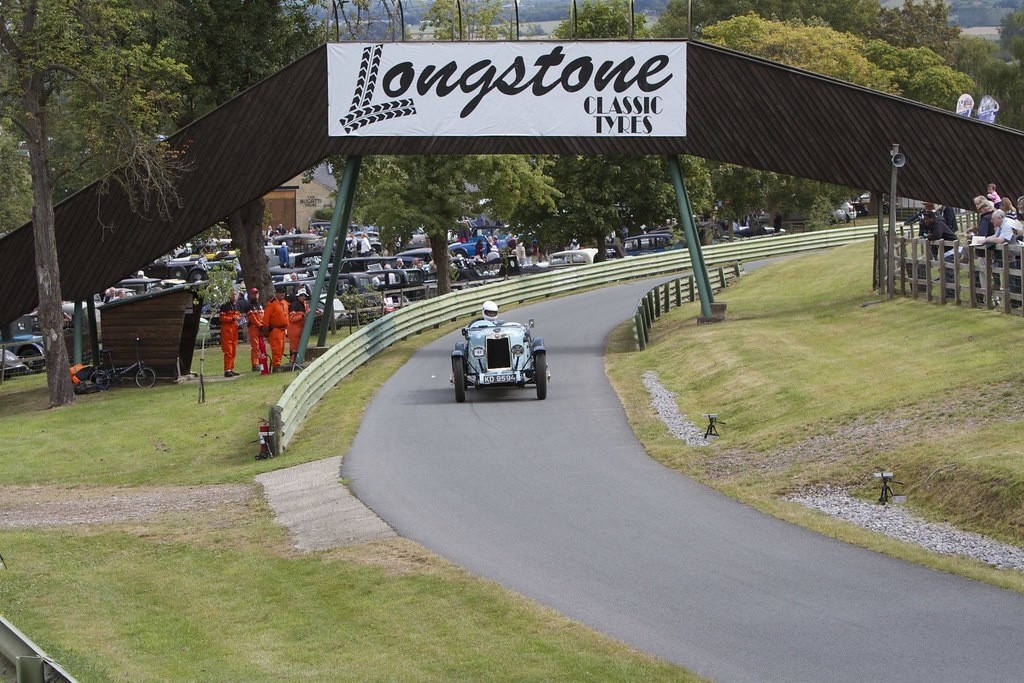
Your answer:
252,366,260,372
1011,302,1020,308
231,371,240,375
225,371,233,377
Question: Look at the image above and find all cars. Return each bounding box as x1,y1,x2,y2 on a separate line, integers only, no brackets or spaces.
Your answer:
0,221,787,372
450,319,550,402
825,194,891,226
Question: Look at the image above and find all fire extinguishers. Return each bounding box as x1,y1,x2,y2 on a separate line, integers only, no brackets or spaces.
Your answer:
258,416,270,452
257,351,271,375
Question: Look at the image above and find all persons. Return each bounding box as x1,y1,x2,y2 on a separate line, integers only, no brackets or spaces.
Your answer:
95,288,125,303
919,202,963,299
475,234,526,271
483,301,498,322
457,221,471,243
478,220,500,234
220,272,321,377
199,254,207,270
385,258,437,303
773,212,782,233
266,224,371,268
967,184,1024,309
139,271,151,288
62,301,71,321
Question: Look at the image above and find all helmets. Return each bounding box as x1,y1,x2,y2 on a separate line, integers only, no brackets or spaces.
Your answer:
482,301,499,321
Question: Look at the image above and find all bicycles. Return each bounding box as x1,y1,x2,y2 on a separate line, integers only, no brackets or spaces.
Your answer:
90,336,157,391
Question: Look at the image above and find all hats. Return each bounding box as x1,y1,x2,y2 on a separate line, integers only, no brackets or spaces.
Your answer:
490,245,498,251
249,288,259,293
296,288,309,297
276,286,285,292
